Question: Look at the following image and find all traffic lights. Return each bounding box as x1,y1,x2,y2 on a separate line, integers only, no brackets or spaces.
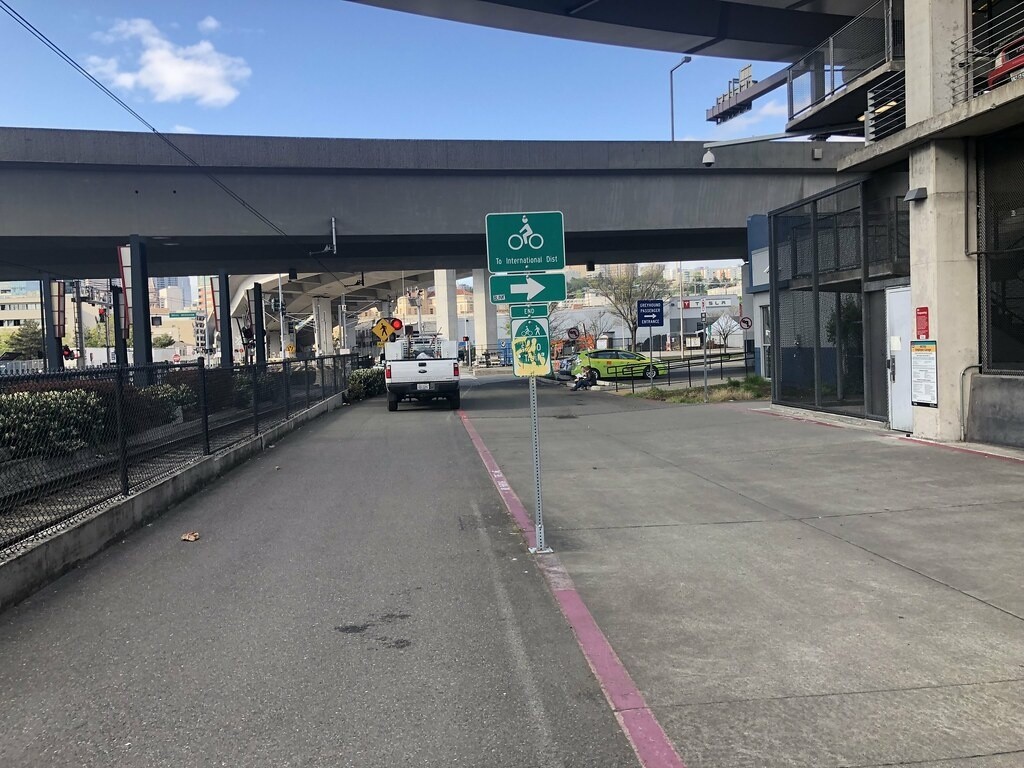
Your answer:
281,301,288,317
287,321,296,335
272,300,280,312
99,308,106,323
391,319,403,330
463,337,470,341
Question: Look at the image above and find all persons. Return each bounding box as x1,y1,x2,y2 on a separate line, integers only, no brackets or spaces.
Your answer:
569,365,596,391
485,350,490,368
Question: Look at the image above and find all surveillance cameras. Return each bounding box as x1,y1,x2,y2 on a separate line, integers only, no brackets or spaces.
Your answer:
325,244,333,251
702,150,715,167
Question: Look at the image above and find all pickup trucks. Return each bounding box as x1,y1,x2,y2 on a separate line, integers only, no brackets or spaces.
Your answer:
379,331,463,411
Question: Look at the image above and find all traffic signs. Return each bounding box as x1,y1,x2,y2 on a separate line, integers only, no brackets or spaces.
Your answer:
636,300,666,327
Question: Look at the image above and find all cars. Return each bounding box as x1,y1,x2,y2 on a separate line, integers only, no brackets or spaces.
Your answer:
571,348,670,379
559,355,577,377
61,345,76,360
